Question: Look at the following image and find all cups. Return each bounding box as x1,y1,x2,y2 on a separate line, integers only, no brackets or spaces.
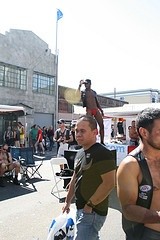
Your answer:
15,141,19,147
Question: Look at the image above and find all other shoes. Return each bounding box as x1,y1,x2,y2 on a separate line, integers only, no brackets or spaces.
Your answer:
34,153,38,155
13,179,19,184
38,153,43,156
0,181,6,186
55,173,60,176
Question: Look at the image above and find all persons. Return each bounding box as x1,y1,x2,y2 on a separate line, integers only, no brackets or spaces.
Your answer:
116,107,160,240
68,124,76,145
76,79,106,146
0,144,20,187
29,124,54,155
5,121,26,148
61,114,116,240
117,118,124,134
52,119,75,171
129,120,139,146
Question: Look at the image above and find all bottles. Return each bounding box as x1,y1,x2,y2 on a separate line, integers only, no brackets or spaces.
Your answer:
66,218,74,240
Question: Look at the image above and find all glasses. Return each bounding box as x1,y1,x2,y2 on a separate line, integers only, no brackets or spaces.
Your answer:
3,148,8,151
58,123,62,124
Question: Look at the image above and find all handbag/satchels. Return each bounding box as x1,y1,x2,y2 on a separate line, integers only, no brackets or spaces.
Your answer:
48,213,75,240
58,142,69,155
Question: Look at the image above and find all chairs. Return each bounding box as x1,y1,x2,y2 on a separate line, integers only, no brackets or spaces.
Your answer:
0,147,45,191
50,157,72,199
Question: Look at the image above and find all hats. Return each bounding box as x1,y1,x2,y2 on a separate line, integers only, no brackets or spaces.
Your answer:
57,119,64,123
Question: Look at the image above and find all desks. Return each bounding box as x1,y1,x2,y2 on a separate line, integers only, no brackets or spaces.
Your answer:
106,144,138,167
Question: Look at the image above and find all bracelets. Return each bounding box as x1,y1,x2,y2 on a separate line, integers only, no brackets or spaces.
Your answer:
87,200,95,208
